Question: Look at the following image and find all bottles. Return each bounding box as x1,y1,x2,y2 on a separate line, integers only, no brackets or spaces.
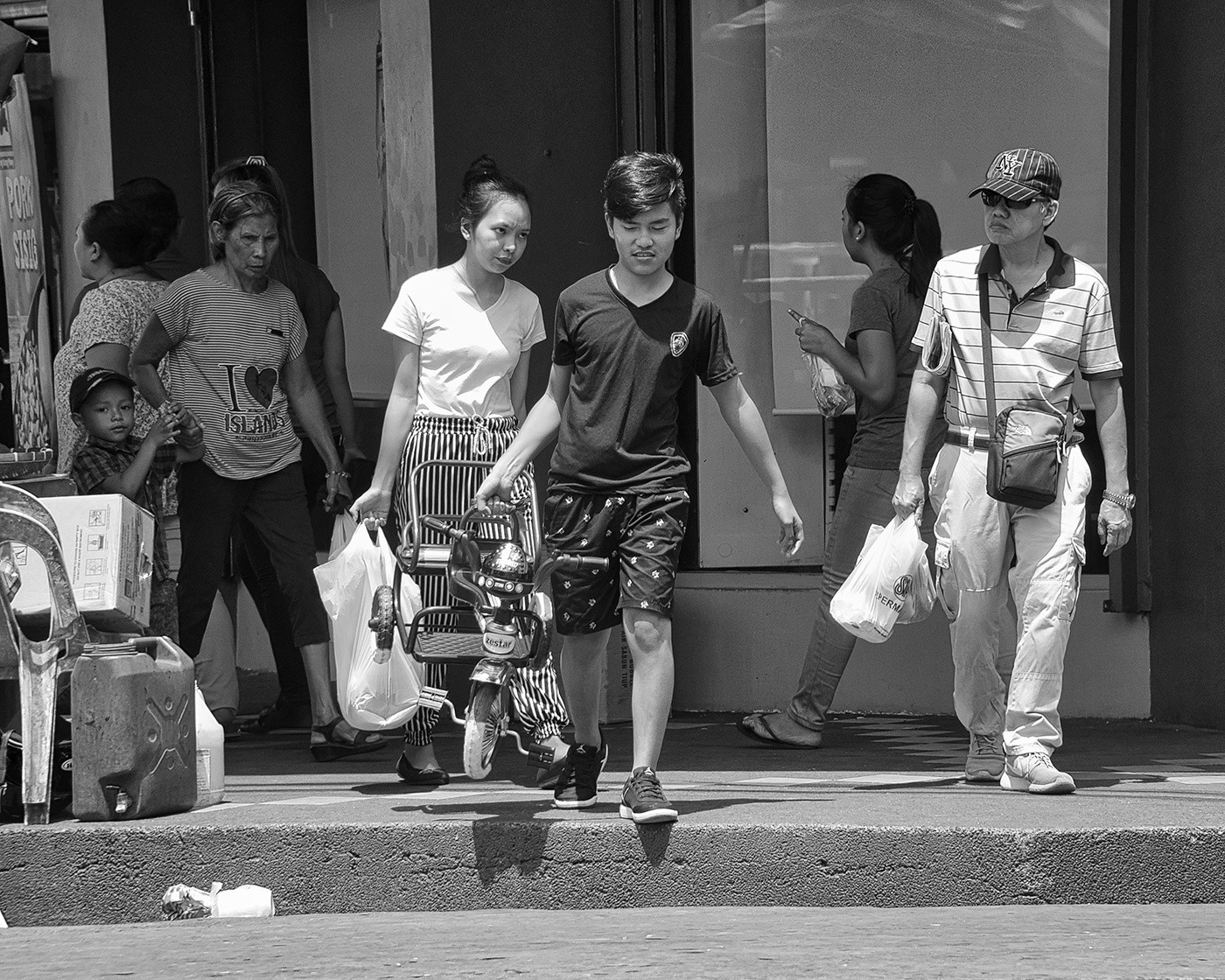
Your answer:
194,677,224,805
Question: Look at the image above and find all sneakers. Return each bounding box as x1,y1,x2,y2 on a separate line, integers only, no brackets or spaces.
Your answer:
554,727,609,808
619,766,678,824
964,705,1005,781
1000,752,1076,794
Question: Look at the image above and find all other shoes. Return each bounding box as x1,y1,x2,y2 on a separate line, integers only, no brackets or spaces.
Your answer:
238,694,311,734
536,754,576,787
395,751,449,784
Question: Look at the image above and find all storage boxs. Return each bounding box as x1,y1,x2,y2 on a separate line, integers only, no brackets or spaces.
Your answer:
7,494,154,627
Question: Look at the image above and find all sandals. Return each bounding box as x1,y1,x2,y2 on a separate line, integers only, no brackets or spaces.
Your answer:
308,718,388,762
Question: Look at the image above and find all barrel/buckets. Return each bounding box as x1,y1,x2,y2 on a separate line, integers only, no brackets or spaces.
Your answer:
69,637,197,822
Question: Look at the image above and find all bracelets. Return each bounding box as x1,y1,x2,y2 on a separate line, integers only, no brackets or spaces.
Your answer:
325,471,352,478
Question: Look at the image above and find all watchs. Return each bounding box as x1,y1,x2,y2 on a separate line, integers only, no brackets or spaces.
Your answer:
1102,490,1136,509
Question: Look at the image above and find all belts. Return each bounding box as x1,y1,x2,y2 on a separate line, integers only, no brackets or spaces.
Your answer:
943,431,1085,449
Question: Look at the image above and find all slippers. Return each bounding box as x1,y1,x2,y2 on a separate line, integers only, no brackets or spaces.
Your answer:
736,712,819,749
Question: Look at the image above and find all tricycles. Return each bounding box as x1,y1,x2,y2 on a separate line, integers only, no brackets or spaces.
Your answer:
366,454,613,782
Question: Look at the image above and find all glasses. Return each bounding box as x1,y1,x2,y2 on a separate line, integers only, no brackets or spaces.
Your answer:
981,189,1049,210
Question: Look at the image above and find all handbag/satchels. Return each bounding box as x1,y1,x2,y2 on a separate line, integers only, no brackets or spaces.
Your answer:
828,511,936,644
986,405,1066,509
312,510,425,731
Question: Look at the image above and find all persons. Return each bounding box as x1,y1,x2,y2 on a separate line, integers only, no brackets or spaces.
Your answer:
53,155,389,763
891,147,1137,794
736,169,1018,749
347,150,805,824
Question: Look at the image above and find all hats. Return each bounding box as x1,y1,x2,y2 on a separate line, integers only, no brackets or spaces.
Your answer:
69,367,137,413
968,147,1062,201
